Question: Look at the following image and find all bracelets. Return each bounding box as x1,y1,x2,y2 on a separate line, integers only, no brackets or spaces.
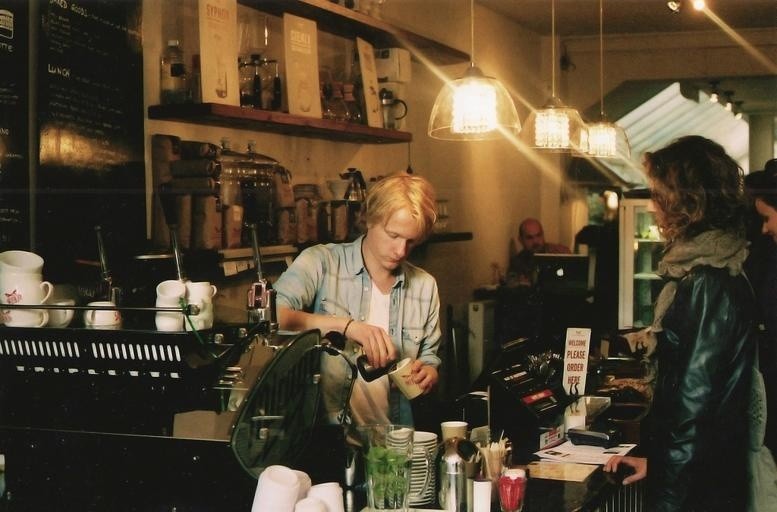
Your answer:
341,320,355,339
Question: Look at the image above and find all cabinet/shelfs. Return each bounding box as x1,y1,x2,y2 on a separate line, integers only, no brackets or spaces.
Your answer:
148,0,474,268
616,188,669,334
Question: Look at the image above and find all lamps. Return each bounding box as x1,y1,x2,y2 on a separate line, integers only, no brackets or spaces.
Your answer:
518,1,591,150
428,0,522,142
572,0,631,160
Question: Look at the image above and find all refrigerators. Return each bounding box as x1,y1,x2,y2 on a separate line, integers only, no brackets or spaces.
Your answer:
617,197,675,333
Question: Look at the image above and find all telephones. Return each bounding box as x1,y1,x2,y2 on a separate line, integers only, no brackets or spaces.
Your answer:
567,424,621,449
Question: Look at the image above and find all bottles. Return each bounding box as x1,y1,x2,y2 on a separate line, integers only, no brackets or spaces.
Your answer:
320,81,329,118
342,84,362,123
160,39,187,103
331,82,350,121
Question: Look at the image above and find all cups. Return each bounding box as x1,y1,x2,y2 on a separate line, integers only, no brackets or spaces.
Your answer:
388,357,429,401
358,421,431,511
1,276,54,306
82,300,121,328
185,307,214,329
440,420,469,443
0,306,49,328
0,248,46,273
48,300,77,331
327,179,351,201
154,312,185,332
157,279,186,303
187,281,217,303
497,473,527,510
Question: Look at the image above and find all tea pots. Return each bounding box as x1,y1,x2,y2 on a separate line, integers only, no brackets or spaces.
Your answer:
380,87,409,129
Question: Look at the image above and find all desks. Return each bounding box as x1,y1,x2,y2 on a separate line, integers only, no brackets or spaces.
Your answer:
490,428,648,512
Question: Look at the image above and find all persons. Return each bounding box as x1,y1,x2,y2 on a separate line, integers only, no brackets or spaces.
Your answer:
743,170,764,206
756,158,777,241
509,219,574,283
603,135,758,512
272,172,442,457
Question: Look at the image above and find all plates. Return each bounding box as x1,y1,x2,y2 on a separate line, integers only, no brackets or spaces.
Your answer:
384,429,438,507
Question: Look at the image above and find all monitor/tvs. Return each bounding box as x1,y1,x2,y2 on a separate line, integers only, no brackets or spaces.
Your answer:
530,253,590,306
